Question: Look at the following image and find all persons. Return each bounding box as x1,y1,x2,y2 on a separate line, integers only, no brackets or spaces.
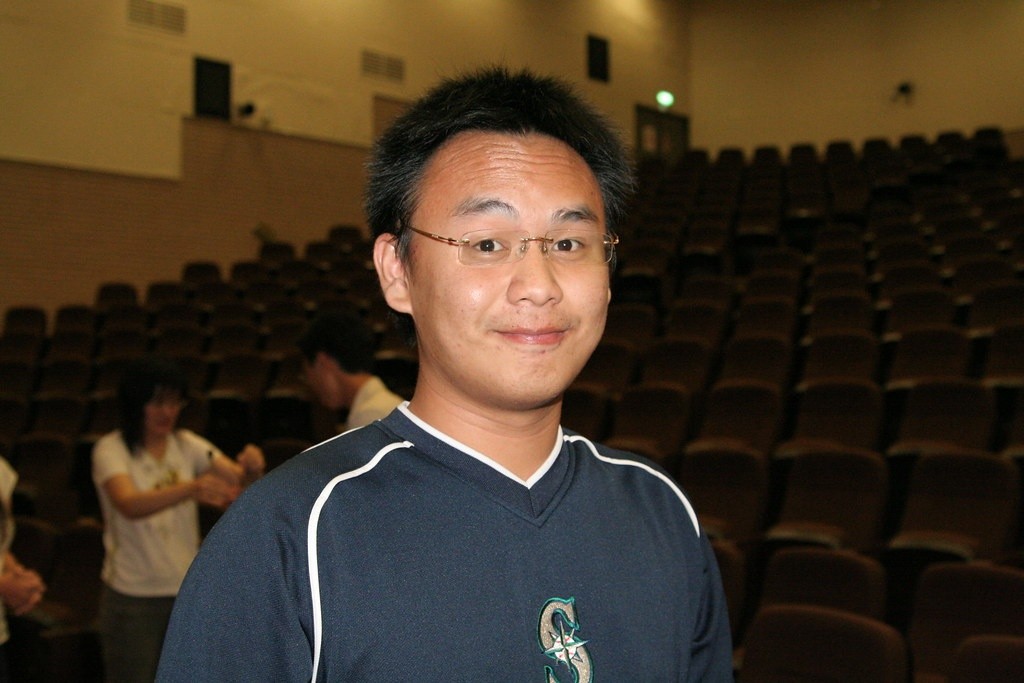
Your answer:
296,311,405,432
153,66,733,683
90,357,266,683
0,456,46,647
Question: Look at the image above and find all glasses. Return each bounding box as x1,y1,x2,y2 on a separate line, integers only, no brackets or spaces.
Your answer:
403,224,620,269
151,397,192,411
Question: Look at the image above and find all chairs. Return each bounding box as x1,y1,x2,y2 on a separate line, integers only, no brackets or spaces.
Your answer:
0,124,1024,683
